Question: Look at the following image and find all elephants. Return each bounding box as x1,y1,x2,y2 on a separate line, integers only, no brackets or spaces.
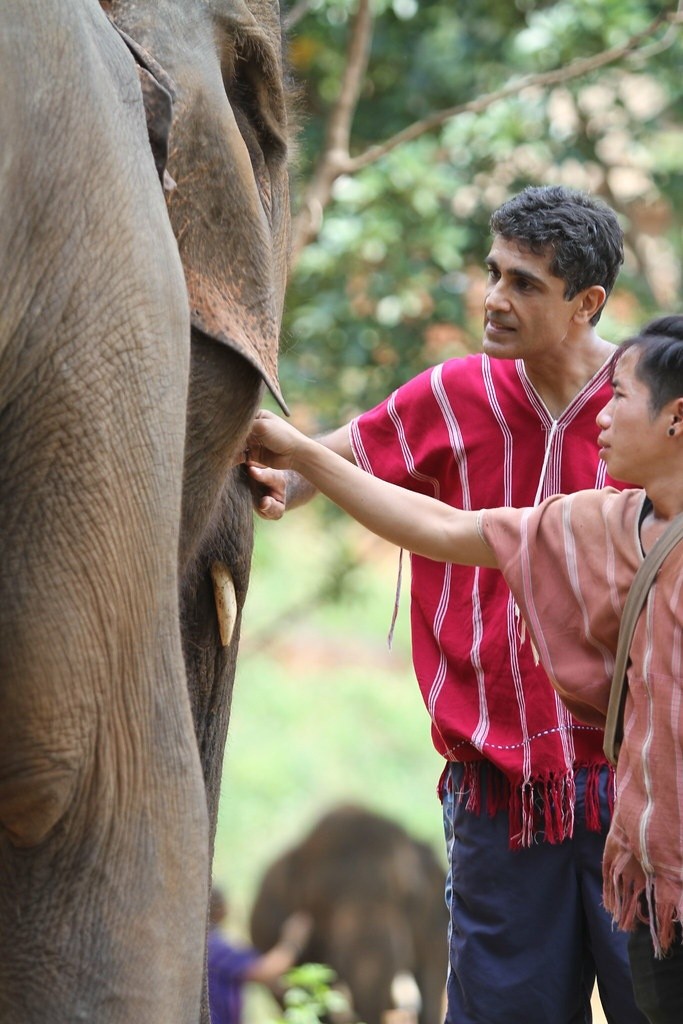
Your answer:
249,806,448,1023
4,4,294,1021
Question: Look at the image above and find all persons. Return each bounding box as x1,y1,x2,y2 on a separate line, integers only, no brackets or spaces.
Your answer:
233,316,683,1023
206,889,311,1024
248,185,650,1024
